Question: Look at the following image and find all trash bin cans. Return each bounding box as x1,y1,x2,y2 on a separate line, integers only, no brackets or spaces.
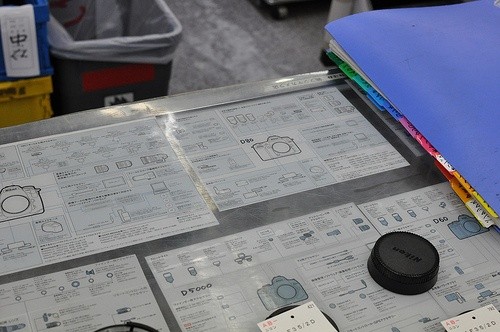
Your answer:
48,0,183,117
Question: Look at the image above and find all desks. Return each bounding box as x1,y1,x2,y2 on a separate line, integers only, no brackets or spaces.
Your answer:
0,67,500,331
